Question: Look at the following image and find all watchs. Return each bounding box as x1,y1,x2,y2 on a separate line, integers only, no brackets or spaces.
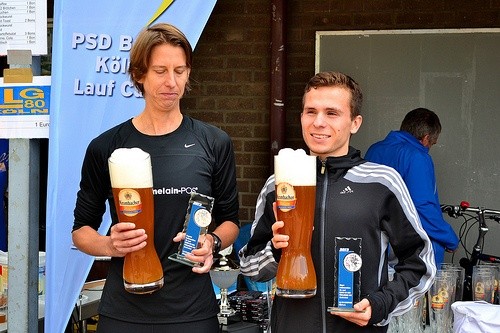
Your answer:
206,232,221,258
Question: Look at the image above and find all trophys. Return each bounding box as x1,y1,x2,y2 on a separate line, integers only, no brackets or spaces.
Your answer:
328,237,365,314
209,244,241,325
168,192,215,267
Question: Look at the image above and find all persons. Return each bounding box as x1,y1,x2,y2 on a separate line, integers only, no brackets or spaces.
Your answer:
364,108,459,270
71,23,241,333
239,71,437,333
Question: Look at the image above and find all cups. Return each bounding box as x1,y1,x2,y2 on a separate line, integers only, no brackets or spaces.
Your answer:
274,147,318,298
108,149,164,295
387,263,500,333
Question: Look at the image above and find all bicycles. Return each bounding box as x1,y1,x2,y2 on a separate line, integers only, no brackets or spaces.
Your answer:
440,201,500,301
